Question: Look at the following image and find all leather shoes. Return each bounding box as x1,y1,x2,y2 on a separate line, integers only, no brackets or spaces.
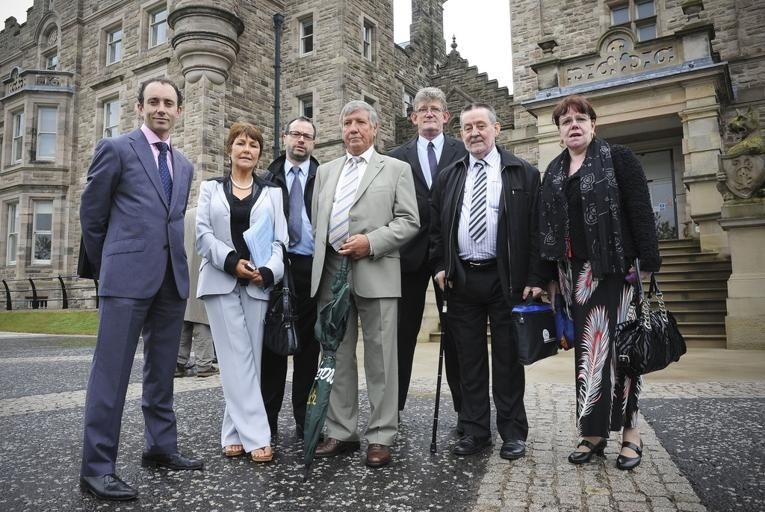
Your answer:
81,472,139,503
365,444,391,466
315,438,361,457
453,435,492,455
499,439,525,459
141,452,204,471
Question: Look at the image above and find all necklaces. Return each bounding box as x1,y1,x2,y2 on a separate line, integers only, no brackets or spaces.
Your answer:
230,175,254,189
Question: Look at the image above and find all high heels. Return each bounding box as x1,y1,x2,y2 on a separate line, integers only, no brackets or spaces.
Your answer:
568,438,607,465
617,440,643,470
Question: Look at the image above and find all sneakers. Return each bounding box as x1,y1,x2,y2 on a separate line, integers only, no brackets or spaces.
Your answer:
175,363,186,377
194,366,219,378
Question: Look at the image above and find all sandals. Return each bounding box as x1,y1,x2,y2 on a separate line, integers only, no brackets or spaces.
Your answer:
226,445,243,456
252,448,274,462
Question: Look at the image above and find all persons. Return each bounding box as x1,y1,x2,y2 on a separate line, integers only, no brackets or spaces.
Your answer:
255,116,325,443
173,207,220,377
194,121,290,463
427,101,545,459
315,101,421,468
387,86,469,425
79,78,204,501
536,95,662,471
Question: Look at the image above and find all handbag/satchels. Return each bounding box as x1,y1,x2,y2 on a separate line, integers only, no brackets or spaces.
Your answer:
614,309,686,376
512,293,559,365
264,286,299,354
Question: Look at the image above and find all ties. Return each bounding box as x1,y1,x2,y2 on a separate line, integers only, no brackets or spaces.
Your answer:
287,165,303,245
329,158,357,252
153,141,173,207
469,161,488,244
428,141,438,180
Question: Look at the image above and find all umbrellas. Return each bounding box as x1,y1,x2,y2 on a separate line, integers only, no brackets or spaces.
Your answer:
302,243,351,481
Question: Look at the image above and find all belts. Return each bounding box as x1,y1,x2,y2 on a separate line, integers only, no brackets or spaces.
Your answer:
461,260,496,271
